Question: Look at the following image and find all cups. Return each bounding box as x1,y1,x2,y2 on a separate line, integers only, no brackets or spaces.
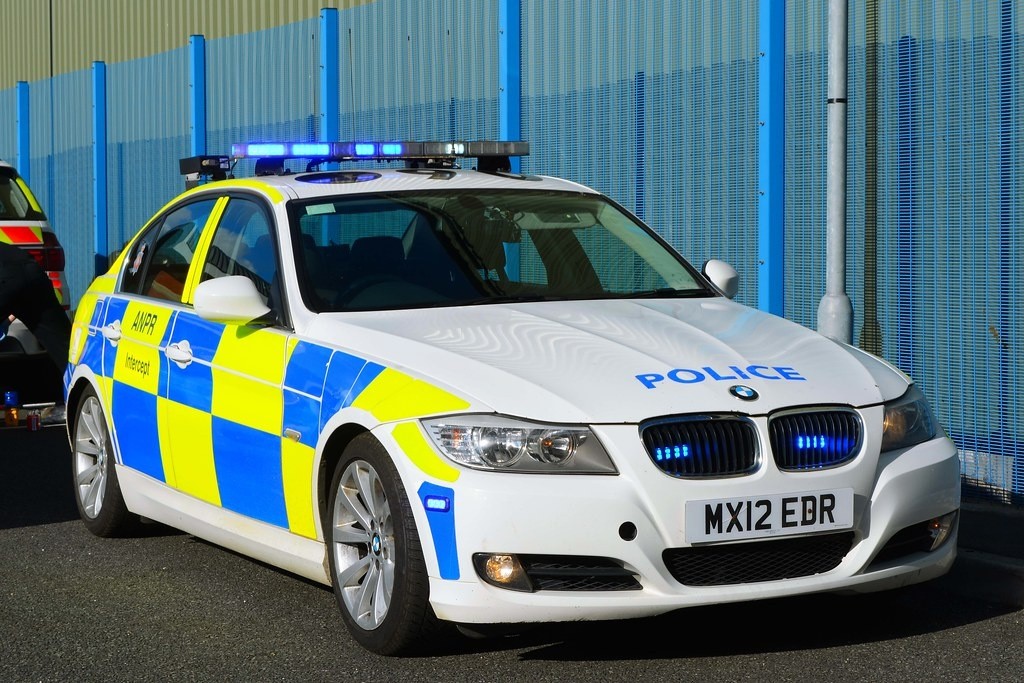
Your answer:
5,391,19,427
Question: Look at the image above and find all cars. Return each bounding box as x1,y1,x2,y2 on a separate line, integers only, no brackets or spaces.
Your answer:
61,141,959,659
0,159,76,430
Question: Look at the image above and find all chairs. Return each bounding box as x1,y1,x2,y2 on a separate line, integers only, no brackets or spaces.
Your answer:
345,236,406,300
